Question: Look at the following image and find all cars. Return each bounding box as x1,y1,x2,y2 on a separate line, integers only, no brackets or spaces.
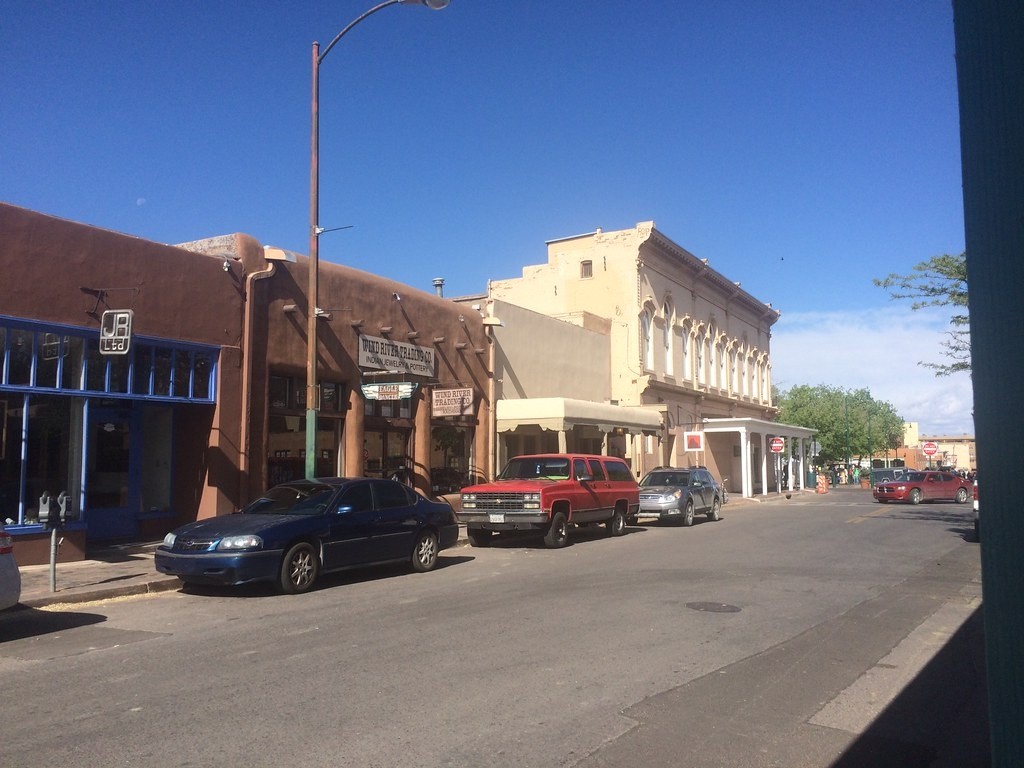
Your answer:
872,468,980,541
155,477,459,595
0,522,21,611
634,466,722,526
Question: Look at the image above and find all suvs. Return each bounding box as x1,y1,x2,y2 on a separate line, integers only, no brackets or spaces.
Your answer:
459,454,641,549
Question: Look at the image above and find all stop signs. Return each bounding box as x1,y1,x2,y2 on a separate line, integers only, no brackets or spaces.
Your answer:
923,442,937,456
768,438,784,453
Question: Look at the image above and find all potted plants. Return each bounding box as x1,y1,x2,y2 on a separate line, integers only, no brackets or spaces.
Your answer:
857,467,871,488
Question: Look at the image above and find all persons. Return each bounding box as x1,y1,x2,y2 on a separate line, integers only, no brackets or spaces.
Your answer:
957,470,972,479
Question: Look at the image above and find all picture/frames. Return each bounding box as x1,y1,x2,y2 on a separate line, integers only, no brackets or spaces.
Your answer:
683,431,705,452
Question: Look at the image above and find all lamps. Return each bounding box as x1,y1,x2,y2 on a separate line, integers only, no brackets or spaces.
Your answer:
483,317,504,337
218,253,230,271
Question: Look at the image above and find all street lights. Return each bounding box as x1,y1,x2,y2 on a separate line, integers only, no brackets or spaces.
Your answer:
306,0,452,478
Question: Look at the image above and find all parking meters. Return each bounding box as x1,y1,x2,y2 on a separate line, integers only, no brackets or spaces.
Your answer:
39,491,72,593
